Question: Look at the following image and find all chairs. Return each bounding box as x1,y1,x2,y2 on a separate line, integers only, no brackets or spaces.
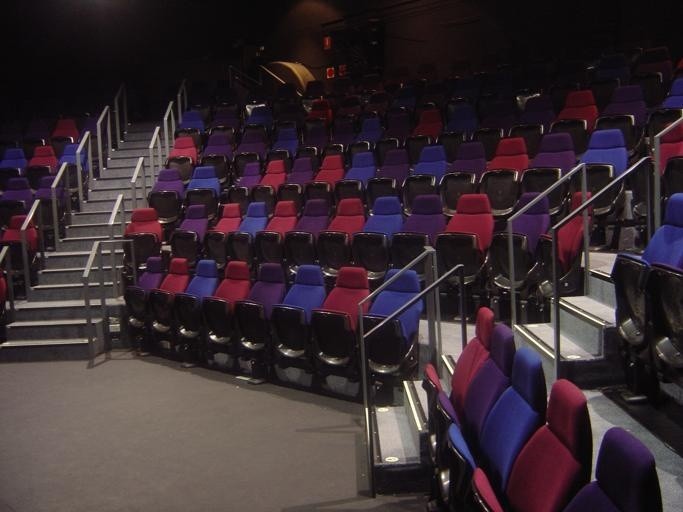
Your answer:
1,101,115,309
